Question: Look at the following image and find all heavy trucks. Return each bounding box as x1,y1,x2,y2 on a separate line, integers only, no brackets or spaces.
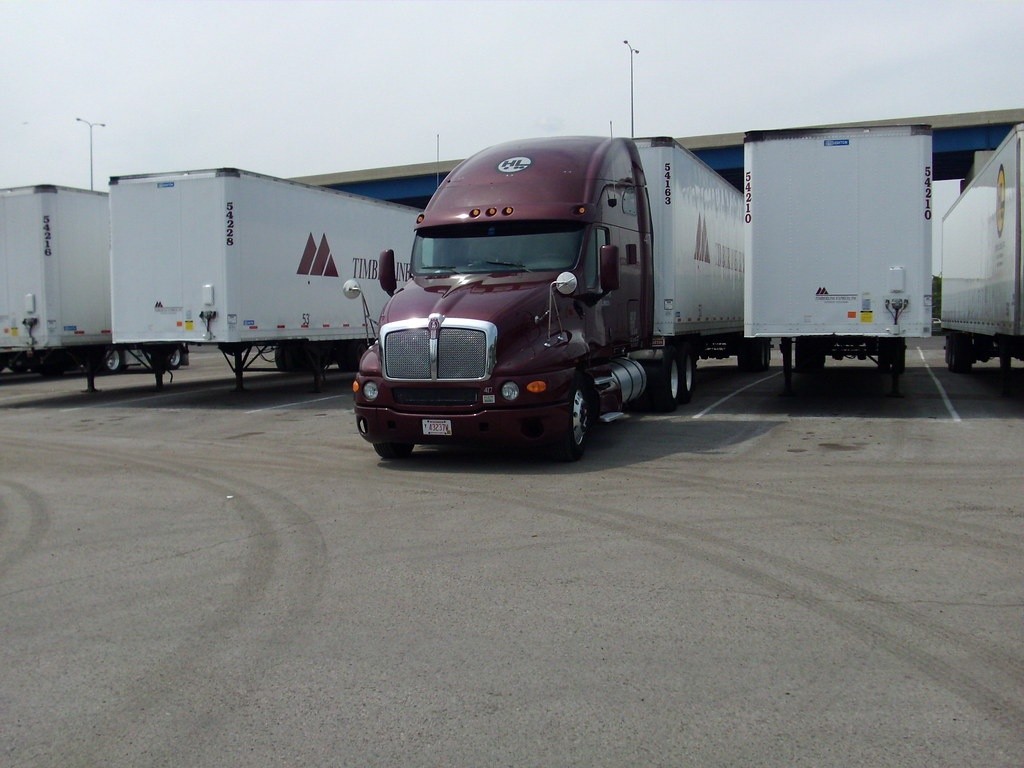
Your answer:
339,140,774,464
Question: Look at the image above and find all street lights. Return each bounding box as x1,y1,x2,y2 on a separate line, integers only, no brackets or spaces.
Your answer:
623,39,640,139
76,118,106,191
